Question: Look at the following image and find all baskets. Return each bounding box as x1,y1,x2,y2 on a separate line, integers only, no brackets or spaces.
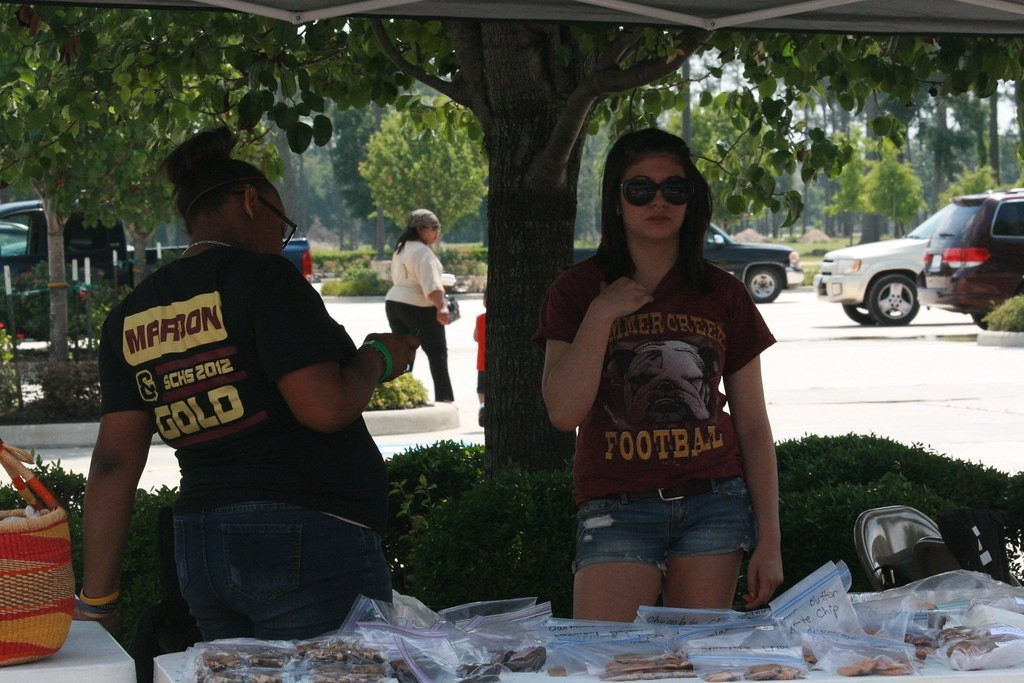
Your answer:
0,442,76,666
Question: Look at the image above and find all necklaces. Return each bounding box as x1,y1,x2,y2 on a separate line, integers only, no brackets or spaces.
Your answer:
182,240,231,255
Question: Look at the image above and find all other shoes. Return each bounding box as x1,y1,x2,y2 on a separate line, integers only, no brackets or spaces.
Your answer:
480,407,486,426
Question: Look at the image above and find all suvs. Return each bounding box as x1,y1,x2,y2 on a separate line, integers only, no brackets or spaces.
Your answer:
916,189,1024,330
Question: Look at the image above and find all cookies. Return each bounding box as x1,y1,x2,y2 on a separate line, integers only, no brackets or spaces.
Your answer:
595,629,938,682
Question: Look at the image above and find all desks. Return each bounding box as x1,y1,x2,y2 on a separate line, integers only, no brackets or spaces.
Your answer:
153,650,1024,683
0,621,137,682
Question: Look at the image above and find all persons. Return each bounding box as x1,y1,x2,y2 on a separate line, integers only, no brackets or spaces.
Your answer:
385,208,454,404
540,128,783,622
473,288,486,427
71,125,420,643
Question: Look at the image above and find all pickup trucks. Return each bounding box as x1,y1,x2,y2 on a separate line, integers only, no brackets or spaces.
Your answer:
0,196,313,289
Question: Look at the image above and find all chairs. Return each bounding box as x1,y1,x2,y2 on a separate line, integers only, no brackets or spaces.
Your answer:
853,505,1023,593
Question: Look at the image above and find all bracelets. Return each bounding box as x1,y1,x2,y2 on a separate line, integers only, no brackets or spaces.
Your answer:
364,340,392,383
79,586,121,620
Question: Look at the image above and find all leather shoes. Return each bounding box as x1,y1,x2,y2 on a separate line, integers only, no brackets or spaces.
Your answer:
591,476,740,500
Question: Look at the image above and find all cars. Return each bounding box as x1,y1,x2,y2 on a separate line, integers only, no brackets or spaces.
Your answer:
704,222,805,304
813,203,952,327
0,221,30,260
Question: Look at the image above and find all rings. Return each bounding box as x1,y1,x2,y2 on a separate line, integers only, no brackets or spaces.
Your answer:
403,363,410,373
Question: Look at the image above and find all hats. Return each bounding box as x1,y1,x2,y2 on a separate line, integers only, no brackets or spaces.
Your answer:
408,208,440,226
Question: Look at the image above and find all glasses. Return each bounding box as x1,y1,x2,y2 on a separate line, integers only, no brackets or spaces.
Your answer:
231,190,296,249
620,175,694,207
